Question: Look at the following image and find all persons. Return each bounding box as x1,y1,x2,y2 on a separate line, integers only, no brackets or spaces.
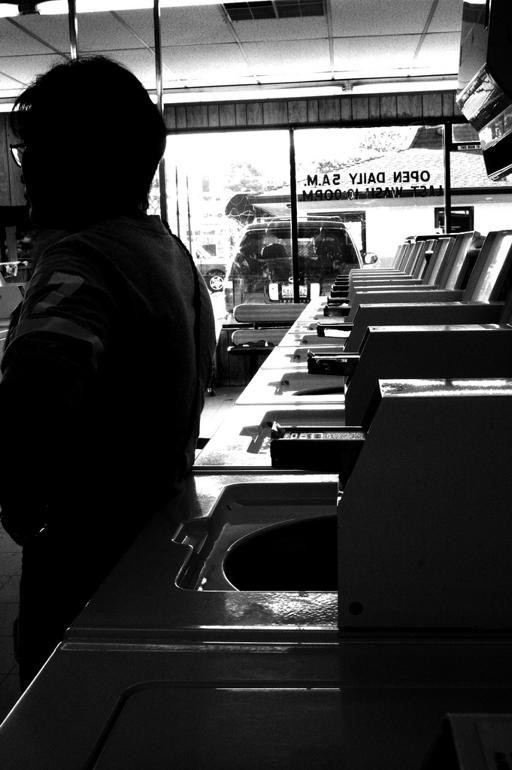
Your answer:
2,51,216,701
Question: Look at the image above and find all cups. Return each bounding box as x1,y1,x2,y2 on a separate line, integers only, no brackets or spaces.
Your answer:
8,141,31,168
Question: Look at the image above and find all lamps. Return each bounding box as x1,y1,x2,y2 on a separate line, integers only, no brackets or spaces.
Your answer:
260,239,342,275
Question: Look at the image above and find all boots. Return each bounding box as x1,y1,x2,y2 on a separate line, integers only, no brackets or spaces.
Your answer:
221,216,378,319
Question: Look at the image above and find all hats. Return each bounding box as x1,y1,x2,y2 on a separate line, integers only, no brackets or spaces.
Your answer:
180,240,231,293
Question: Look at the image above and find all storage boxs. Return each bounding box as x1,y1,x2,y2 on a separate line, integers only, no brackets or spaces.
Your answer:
227,303,307,390
